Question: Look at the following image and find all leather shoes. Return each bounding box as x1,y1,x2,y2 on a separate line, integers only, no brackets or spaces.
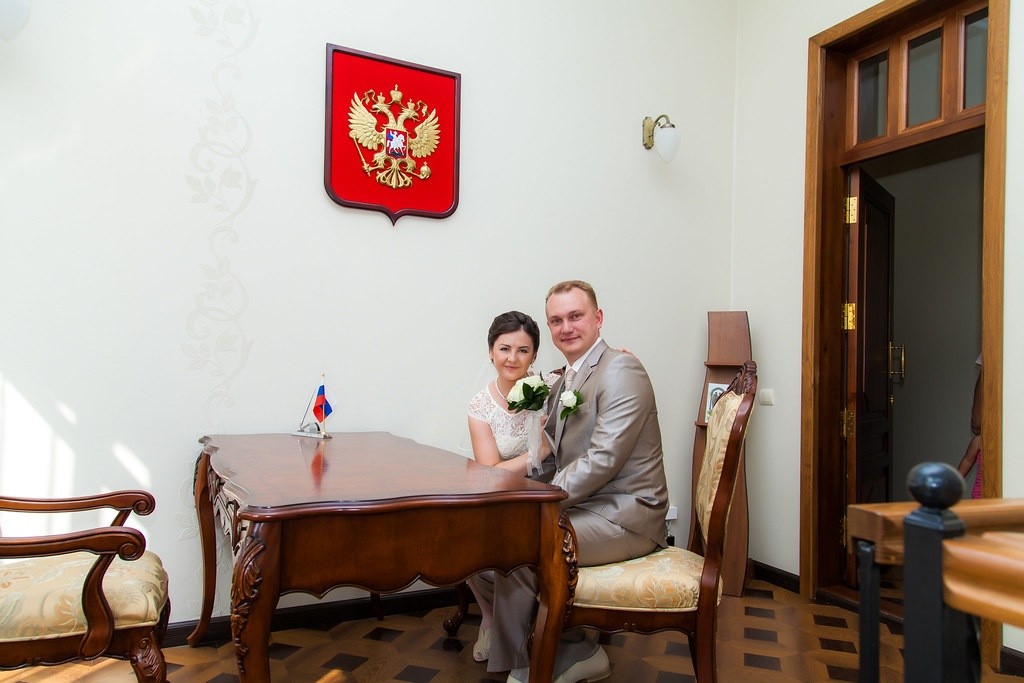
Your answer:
554,645,610,683
506,667,530,683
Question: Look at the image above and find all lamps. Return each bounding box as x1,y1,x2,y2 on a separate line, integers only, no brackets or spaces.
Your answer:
642,113,674,149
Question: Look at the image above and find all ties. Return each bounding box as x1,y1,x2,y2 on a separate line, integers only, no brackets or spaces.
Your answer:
560,369,577,416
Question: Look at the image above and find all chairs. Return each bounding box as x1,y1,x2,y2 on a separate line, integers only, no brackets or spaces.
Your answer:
0,490,171,683
442,578,483,635
528,359,759,683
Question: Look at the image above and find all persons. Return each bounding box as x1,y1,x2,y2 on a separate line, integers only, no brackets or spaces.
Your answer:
970,352,982,435
958,434,982,500
466,311,630,661
486,280,669,683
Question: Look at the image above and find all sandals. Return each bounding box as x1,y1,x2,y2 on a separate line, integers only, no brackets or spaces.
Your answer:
473,618,494,661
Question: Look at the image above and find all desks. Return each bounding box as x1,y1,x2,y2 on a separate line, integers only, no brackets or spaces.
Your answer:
185,431,581,683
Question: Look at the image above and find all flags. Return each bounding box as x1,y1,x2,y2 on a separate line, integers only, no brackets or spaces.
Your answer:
312,378,332,423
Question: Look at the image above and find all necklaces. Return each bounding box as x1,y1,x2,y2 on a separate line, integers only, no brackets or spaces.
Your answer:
495,376,508,402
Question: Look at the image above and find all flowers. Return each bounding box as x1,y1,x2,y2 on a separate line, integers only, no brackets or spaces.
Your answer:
559,389,583,420
506,370,552,413
